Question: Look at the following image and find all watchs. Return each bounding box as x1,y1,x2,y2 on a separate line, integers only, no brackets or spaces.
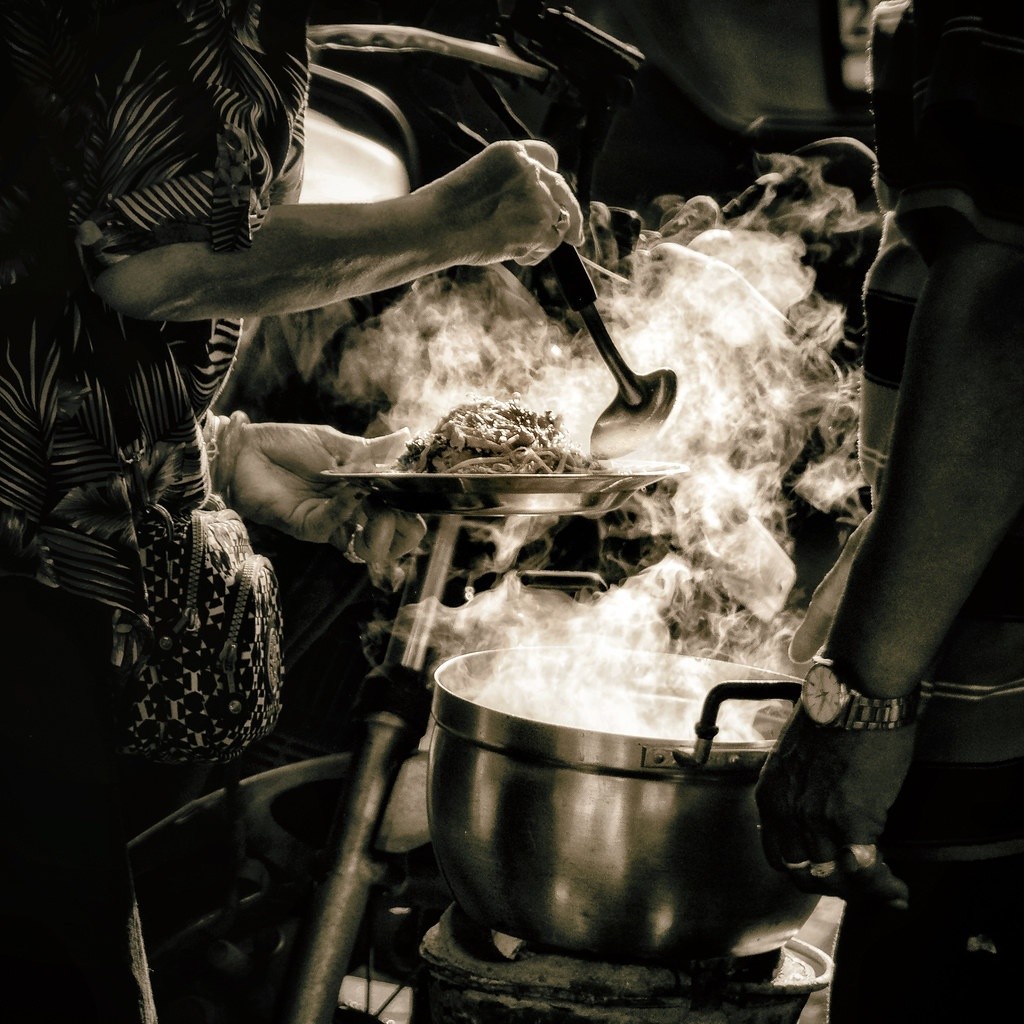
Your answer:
797,663,925,735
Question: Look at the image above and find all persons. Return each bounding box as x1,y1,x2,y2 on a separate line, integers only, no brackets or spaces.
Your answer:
751,0,1020,1024
0,0,594,1022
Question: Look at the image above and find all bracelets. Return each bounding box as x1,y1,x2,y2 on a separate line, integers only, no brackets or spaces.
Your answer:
198,409,245,493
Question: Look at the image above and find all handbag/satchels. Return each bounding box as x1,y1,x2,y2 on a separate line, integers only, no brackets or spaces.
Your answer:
112,497,283,783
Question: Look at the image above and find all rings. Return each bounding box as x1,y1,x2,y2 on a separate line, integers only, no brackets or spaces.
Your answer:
553,205,572,235
342,523,370,565
777,855,811,869
809,859,841,878
840,843,878,870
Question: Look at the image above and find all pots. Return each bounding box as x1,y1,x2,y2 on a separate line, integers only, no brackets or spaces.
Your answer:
428,642,826,964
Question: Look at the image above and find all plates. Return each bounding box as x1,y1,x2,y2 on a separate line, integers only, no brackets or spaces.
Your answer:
322,463,683,516
